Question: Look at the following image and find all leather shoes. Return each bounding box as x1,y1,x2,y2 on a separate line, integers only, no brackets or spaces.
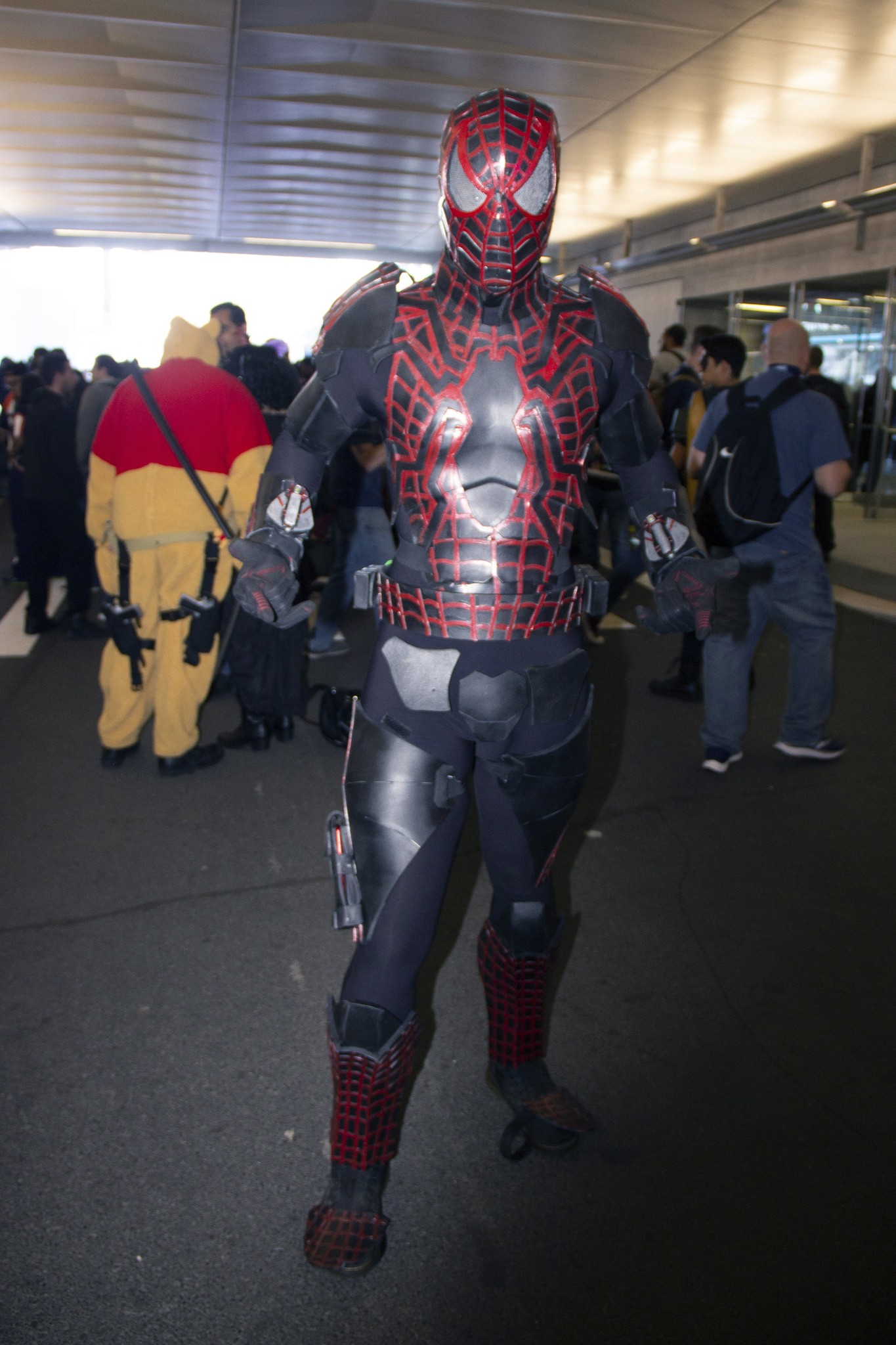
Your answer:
157,743,223,775
100,739,144,766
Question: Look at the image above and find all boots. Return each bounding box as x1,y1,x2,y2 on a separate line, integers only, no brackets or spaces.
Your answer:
319,690,351,748
221,706,270,750
268,704,294,741
477,913,599,1160
305,994,436,1271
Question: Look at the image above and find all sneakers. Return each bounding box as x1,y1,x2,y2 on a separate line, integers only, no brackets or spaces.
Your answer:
774,733,847,759
702,745,743,773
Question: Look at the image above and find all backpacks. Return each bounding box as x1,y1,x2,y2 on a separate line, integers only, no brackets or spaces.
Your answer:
692,376,814,547
662,349,690,379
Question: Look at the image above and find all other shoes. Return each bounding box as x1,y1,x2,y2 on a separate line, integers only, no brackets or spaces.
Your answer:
24,605,59,633
648,664,756,702
63,612,107,640
4,576,28,586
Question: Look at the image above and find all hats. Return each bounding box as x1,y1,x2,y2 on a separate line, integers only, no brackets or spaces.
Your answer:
701,334,745,367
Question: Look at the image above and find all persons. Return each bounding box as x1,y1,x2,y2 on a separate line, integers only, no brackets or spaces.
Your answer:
0,298,896,776
227,89,743,1270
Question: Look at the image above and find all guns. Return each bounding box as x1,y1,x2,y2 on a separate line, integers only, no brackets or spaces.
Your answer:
183,596,216,648
324,809,362,935
101,601,143,655
